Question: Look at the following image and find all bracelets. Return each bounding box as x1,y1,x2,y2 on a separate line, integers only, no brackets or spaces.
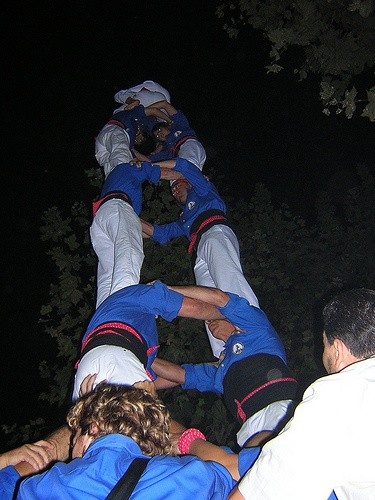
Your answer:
175,426,205,455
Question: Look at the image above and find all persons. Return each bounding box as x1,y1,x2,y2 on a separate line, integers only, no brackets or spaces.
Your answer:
227,287,375,499
1,74,337,500
